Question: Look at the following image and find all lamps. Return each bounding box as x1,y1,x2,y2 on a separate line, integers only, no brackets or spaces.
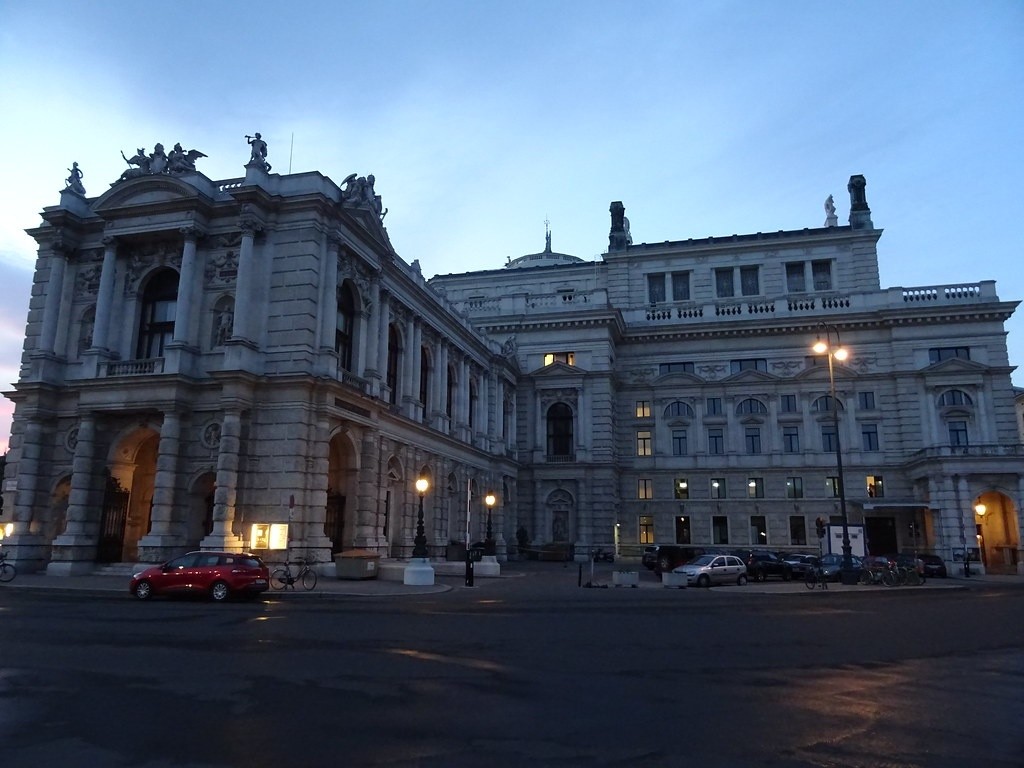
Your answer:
975,497,987,518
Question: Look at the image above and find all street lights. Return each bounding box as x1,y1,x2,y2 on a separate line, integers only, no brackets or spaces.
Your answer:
404,474,434,585
480,493,500,563
811,319,861,586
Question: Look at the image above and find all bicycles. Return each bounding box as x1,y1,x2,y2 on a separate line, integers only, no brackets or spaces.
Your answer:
804,565,824,589
270,556,318,590
0,550,18,583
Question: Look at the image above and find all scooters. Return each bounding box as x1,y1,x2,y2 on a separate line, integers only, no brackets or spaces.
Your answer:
592,548,614,563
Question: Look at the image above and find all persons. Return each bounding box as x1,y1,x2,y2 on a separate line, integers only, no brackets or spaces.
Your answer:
86,322,93,345
68,162,83,183
345,175,379,213
917,559,926,583
120,143,196,181
216,305,232,346
248,133,267,162
864,538,870,556
515,526,528,557
748,557,752,564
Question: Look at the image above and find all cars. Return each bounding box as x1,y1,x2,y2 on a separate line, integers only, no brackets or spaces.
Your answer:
642,543,947,585
671,554,748,588
128,550,271,603
